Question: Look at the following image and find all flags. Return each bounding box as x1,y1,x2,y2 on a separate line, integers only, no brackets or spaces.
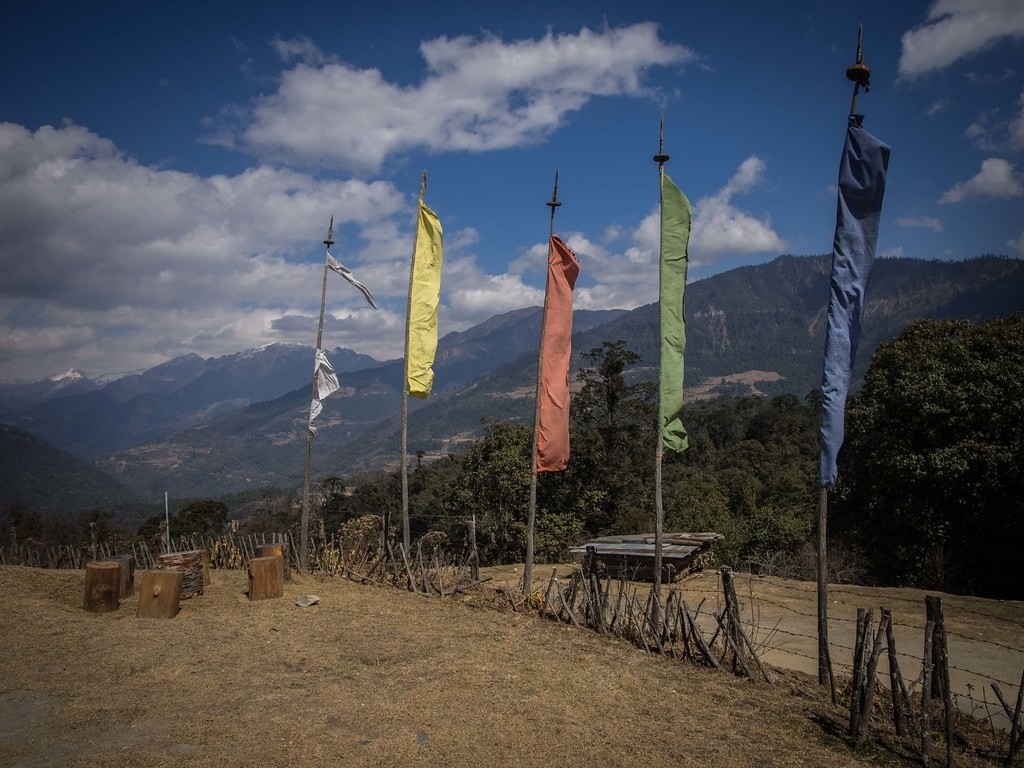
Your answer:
535,234,581,470
817,114,892,487
405,196,444,401
658,171,690,457
